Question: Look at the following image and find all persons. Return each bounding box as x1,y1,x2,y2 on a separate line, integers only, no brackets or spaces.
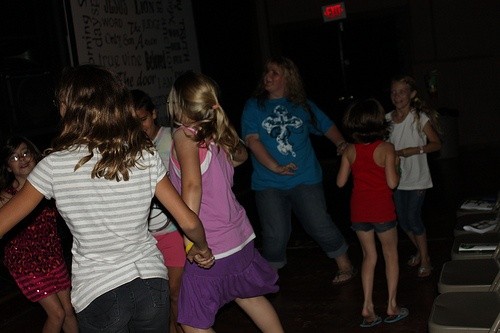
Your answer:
0,64,215,333
131,89,186,333
241,54,355,284
1,136,78,333
335,99,410,328
166,72,284,333
385,75,447,277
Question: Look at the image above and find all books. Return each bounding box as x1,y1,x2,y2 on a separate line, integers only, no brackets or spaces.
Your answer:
463,219,495,234
460,242,497,251
460,198,499,211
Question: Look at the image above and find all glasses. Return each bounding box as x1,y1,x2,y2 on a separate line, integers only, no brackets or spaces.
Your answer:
137,114,152,127
7,149,32,162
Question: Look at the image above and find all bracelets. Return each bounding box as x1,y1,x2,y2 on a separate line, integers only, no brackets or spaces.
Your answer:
336,142,350,148
185,241,194,255
419,145,424,154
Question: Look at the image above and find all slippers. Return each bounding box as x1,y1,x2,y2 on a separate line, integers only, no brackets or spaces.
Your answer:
359,315,382,327
417,264,433,277
331,271,358,286
384,307,409,323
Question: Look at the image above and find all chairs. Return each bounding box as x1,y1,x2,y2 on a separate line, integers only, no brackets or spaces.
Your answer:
428,191,500,333
